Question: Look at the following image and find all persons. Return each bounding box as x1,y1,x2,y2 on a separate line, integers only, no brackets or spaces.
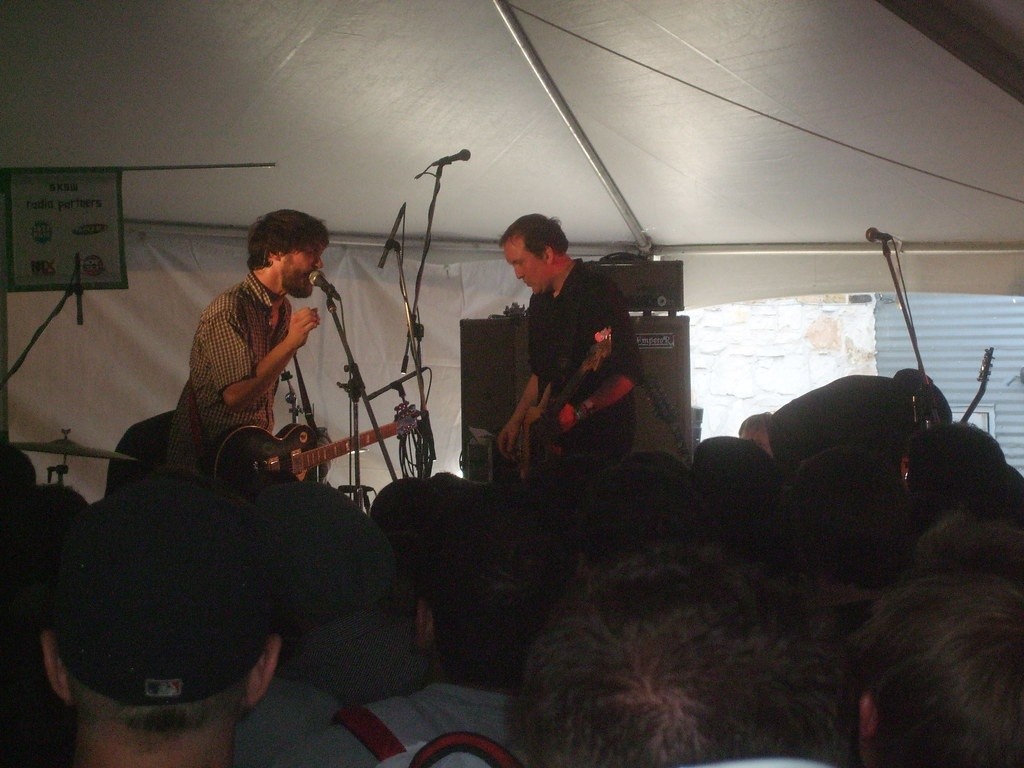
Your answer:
0,442,554,768
164,208,330,488
546,374,1024,768
496,213,645,481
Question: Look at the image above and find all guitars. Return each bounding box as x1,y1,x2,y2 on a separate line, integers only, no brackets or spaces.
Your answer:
210,401,423,480
514,326,616,480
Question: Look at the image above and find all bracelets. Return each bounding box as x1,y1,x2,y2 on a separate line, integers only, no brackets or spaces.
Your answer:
571,402,589,420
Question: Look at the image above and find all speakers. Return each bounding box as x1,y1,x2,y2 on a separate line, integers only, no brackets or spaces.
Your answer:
460,316,535,487
631,314,694,470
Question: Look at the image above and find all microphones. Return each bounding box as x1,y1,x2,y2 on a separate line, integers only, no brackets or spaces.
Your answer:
309,270,342,302
337,485,374,493
367,367,427,401
865,228,894,242
75,253,84,325
378,202,406,269
430,149,471,166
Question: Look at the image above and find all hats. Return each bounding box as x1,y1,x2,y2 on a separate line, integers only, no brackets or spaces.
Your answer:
52,487,255,707
244,482,396,612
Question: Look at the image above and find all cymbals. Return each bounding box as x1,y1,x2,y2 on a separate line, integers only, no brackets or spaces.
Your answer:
5,439,138,462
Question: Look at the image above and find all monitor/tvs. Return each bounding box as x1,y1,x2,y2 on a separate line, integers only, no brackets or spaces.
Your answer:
950,406,995,439
